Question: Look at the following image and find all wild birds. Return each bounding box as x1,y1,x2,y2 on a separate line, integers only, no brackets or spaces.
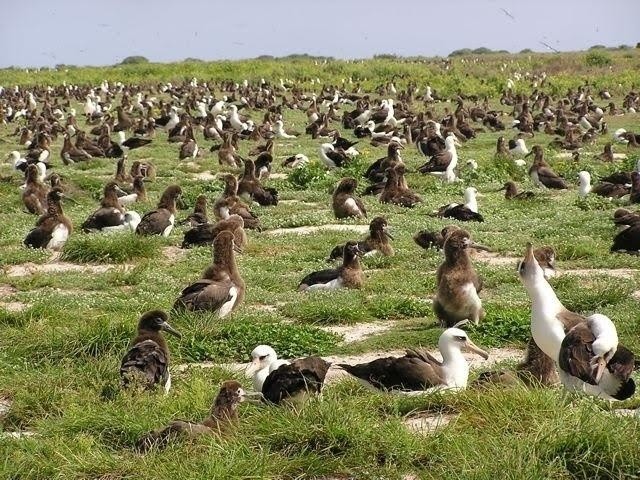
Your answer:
0,70,640,455
